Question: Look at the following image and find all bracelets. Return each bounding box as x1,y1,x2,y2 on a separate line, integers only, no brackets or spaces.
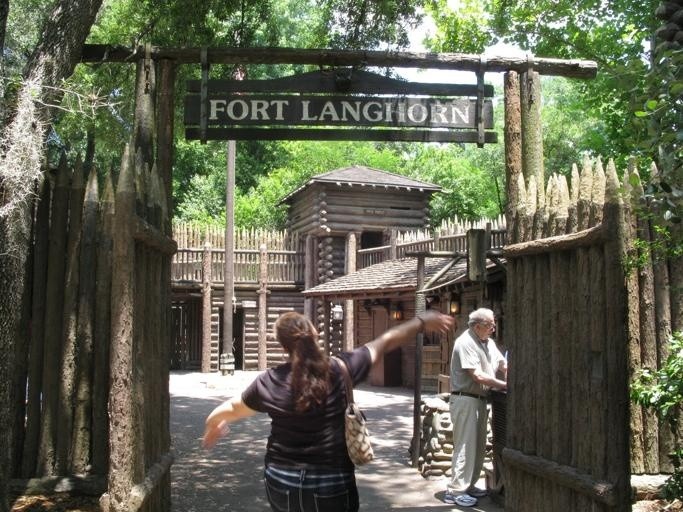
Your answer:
416,316,424,333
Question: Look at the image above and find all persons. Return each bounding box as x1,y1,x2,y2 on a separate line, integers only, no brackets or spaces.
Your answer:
443,307,507,506
202,310,455,511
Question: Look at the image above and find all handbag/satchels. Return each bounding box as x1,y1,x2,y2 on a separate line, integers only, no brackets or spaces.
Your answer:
344,402,376,465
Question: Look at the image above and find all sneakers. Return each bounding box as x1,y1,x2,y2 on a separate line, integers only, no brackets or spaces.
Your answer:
468,486,488,497
444,490,478,507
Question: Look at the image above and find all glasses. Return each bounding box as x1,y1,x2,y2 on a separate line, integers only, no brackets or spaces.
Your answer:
480,320,496,330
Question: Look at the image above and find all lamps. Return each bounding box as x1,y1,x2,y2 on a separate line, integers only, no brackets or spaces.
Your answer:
332,304,343,321
450,294,461,316
389,301,405,321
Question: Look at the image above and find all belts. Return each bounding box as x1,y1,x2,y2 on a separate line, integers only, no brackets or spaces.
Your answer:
451,391,487,400
